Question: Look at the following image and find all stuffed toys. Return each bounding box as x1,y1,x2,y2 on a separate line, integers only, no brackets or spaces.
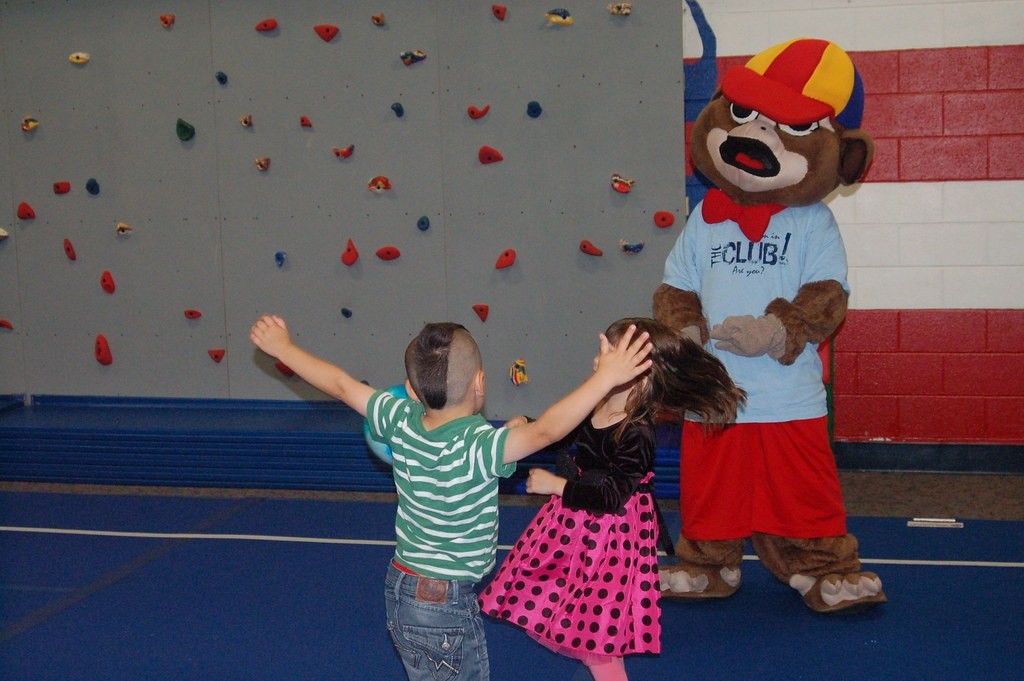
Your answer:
644,39,891,615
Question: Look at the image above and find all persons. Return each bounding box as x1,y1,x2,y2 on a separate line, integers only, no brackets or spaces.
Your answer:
249,314,653,681
478,317,750,681
